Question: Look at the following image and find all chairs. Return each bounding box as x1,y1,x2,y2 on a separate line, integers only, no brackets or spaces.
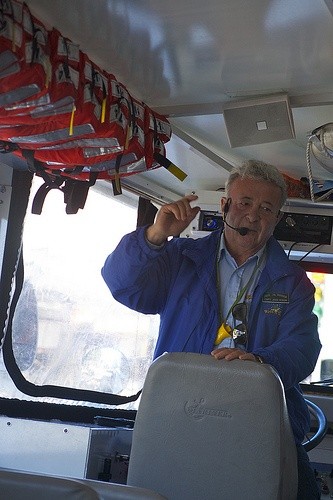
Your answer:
124,351,300,499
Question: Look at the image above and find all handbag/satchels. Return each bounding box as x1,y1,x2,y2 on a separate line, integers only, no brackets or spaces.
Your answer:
35,98,146,185
0,15,50,109
0,52,108,150
0,0,36,92
16,73,131,167
60,110,173,180
0,28,78,127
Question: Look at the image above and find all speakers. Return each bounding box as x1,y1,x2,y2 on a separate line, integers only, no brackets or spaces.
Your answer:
223,92,296,148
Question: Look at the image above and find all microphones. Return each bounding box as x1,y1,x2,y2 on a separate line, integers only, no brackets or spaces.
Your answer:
223,202,248,236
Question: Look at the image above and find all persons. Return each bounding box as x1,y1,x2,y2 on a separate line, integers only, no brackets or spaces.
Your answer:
100,158,323,500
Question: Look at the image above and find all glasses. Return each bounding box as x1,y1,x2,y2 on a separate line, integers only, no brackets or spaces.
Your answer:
231,302,251,351
